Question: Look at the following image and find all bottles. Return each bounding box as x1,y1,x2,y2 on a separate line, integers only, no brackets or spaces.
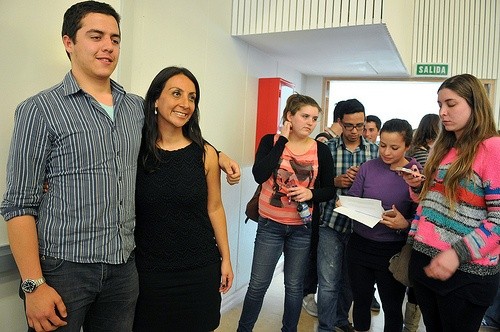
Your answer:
297,202,313,230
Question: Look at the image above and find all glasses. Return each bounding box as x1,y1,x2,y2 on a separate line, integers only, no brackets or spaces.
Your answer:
341,121,363,131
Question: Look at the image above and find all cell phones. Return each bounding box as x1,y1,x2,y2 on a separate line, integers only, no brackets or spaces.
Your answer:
383,209,396,218
390,166,421,177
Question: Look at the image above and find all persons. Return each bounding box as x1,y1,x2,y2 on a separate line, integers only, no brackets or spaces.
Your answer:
398,74,500,332
311,99,380,332
335,119,424,332
302,101,440,332
237,91,338,332
43,67,233,332
0,0,241,332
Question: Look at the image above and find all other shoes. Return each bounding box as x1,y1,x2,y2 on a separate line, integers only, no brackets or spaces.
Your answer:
318,321,356,332
302,296,318,317
370,296,380,312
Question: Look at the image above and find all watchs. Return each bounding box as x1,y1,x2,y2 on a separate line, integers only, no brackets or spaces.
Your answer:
21,277,46,293
